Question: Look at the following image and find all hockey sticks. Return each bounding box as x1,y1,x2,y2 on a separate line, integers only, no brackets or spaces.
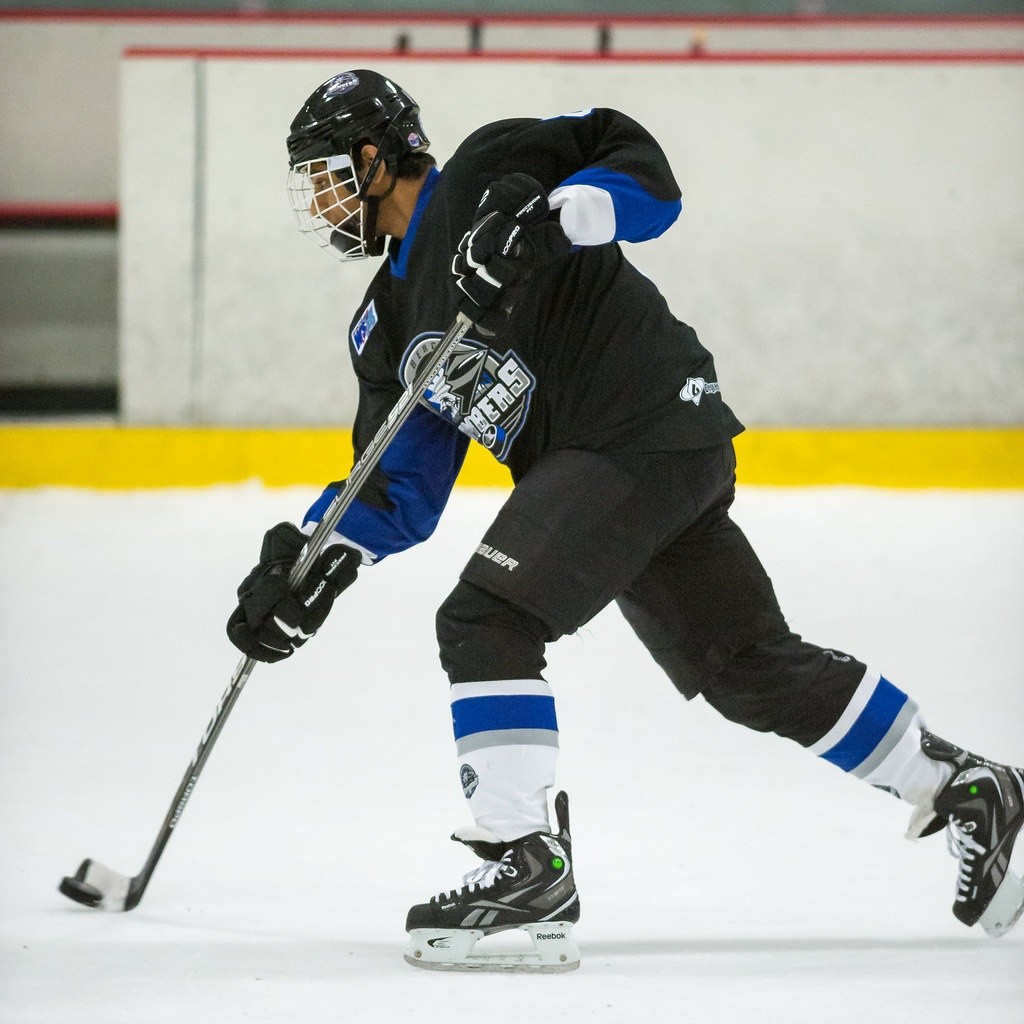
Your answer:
71,295,485,915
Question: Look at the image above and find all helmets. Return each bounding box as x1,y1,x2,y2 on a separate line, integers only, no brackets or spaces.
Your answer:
285,69,434,164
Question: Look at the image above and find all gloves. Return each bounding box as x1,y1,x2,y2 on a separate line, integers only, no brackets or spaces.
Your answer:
448,174,550,337
227,521,362,665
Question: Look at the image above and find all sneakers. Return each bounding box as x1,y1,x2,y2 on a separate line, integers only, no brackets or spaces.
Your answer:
904,730,1024,938
403,790,584,975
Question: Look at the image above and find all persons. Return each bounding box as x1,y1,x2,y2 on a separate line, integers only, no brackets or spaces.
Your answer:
226,67,1024,971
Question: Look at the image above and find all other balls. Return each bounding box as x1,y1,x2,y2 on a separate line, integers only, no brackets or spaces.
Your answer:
58,877,103,908
